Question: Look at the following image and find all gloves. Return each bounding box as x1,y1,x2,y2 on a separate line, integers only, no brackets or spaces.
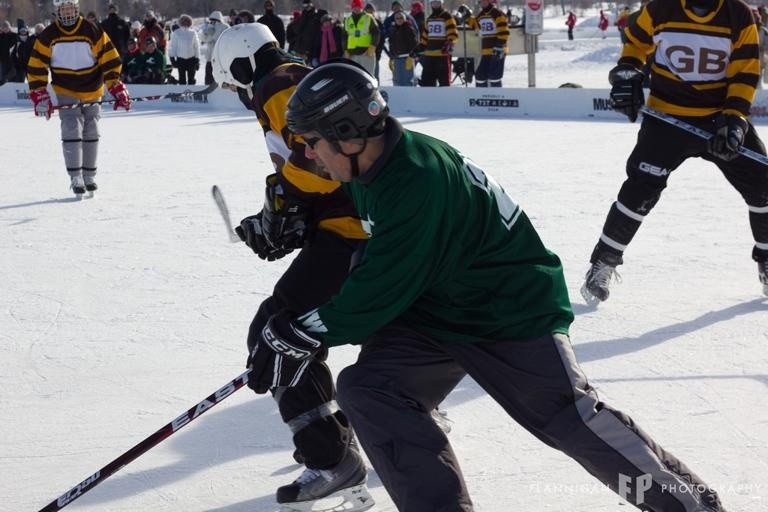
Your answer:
608,64,644,122
459,5,473,18
30,88,55,120
237,216,293,261
261,176,312,248
389,58,394,71
407,57,414,70
108,81,131,112
707,121,745,162
492,41,505,60
245,310,321,393
409,45,423,58
248,300,287,355
442,40,455,53
368,44,376,57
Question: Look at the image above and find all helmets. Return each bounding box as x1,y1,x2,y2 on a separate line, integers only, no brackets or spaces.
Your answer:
126,38,139,48
430,0,443,4
285,64,390,159
145,37,156,48
479,0,497,3
54,1,80,27
211,23,280,89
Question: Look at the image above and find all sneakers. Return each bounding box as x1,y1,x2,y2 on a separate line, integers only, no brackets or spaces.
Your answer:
585,251,623,301
70,175,86,193
277,441,367,503
757,259,768,284
430,406,455,433
83,178,99,192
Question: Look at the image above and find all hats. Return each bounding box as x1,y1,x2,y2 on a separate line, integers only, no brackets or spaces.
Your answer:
109,3,118,10
365,3,376,12
179,15,192,27
17,18,28,33
209,12,224,24
303,0,313,7
351,0,364,10
320,14,334,24
294,9,302,16
87,10,97,17
410,1,424,16
395,12,407,21
1,20,12,32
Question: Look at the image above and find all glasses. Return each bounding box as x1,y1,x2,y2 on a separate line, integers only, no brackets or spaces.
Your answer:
396,17,403,20
303,136,322,150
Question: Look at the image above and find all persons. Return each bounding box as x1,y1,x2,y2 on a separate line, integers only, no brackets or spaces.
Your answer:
229,9,237,26
158,17,179,42
458,4,479,31
597,9,609,39
565,11,576,41
0,20,16,83
614,6,630,32
139,10,166,54
130,21,142,36
235,10,255,24
409,2,425,32
246,63,698,511
168,14,200,85
212,22,374,512
476,0,510,87
26,24,46,56
417,0,458,87
582,0,768,308
201,11,230,85
25,1,130,201
612,6,632,44
86,12,96,21
257,0,285,49
312,15,344,67
364,3,385,87
598,10,610,38
381,2,420,67
342,0,380,76
382,13,420,86
293,1,328,47
101,4,130,60
16,19,29,83
286,9,302,53
121,36,145,84
143,37,168,84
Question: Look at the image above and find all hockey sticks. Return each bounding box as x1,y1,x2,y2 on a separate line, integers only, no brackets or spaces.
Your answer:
52,82,218,109
211,185,242,242
